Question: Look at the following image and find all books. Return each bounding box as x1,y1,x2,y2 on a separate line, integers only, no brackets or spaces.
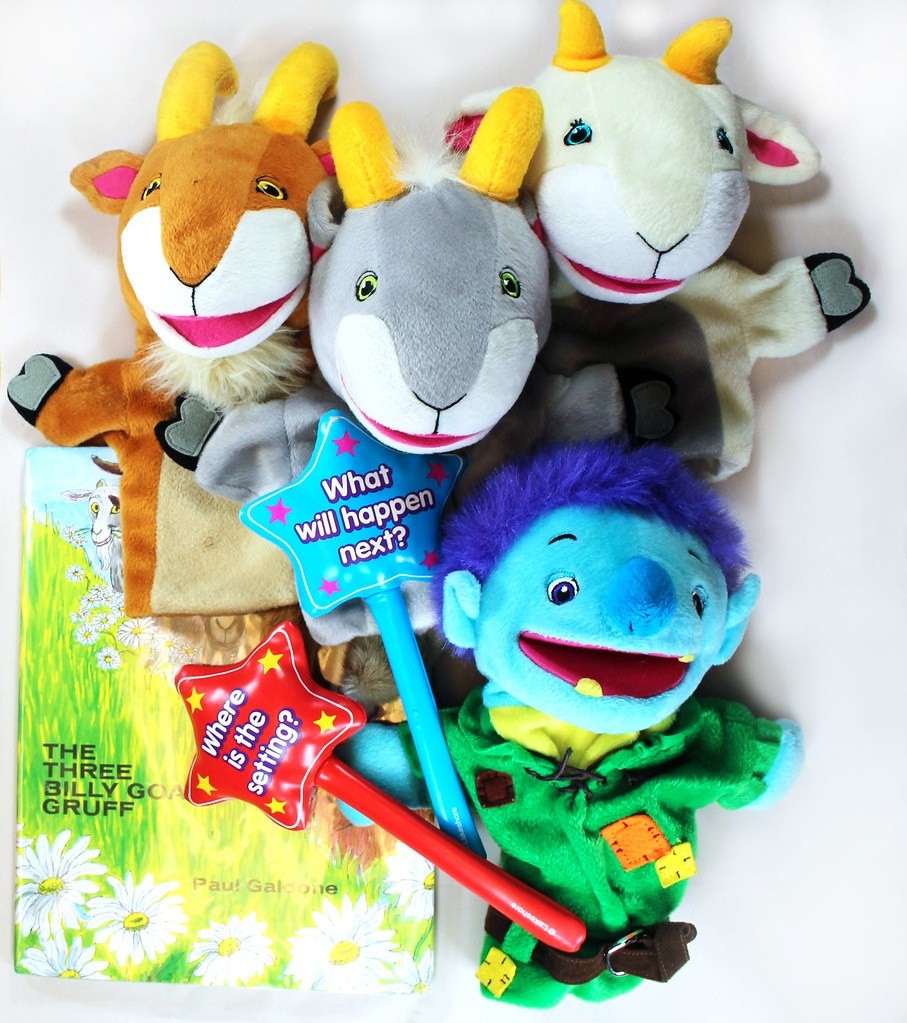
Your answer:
15,446,437,992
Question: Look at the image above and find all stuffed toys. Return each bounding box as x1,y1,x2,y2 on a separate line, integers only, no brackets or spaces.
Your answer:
446,0,872,484
334,434,804,1010
7,39,335,617
154,87,553,643
